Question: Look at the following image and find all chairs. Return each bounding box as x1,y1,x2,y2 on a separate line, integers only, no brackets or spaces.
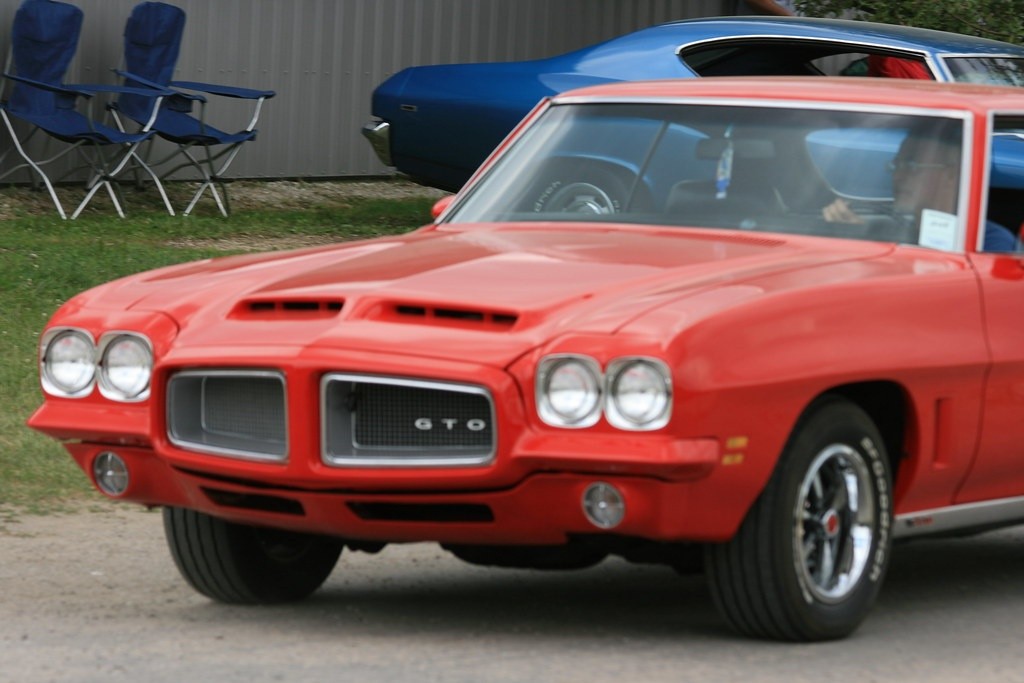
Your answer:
0,0,179,222
85,2,278,218
670,176,792,218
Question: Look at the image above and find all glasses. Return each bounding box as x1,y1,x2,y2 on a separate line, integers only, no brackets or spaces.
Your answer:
887,158,954,177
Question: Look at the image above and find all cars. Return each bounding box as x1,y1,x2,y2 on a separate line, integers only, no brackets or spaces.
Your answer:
22,78,1024,646
356,13,1024,222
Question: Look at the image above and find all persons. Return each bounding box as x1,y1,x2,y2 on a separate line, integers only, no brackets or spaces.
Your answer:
822,122,1024,254
